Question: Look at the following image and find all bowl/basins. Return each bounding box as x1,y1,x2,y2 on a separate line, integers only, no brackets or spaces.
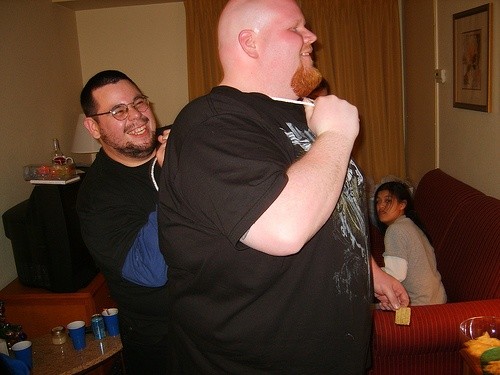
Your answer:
458,315,500,364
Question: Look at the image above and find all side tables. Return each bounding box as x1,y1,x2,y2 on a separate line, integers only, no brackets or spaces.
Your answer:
461,348,483,375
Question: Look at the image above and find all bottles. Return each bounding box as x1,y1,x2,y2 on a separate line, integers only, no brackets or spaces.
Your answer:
51,327,67,345
51,137,63,166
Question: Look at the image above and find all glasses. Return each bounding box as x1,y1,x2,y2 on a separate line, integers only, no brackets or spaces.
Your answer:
87,95,150,120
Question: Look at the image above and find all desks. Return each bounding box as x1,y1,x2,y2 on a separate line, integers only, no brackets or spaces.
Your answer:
9,332,127,375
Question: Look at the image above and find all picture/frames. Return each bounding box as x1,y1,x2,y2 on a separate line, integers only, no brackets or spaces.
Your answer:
453,3,493,113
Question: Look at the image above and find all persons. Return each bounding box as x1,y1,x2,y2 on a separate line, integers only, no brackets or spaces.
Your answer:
157,0,409,375
307,78,331,100
77,69,179,375
373,182,447,305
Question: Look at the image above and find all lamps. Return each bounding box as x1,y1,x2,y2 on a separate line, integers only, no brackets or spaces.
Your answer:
71,113,102,163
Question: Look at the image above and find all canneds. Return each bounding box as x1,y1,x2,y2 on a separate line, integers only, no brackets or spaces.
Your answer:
91,314,106,340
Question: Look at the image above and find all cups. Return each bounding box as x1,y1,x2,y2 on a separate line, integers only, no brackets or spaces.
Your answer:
91,314,107,340
22,162,76,181
101,307,120,337
65,320,87,350
11,340,33,371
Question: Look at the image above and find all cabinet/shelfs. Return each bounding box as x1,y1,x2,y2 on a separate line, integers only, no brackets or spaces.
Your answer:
0,274,118,339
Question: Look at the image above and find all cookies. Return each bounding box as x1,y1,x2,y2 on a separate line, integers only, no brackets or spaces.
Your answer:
395,306,410,325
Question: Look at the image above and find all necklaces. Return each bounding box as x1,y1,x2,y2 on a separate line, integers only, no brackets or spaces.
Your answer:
273,97,314,106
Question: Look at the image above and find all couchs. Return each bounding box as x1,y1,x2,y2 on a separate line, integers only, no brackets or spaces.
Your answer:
368,168,500,375
